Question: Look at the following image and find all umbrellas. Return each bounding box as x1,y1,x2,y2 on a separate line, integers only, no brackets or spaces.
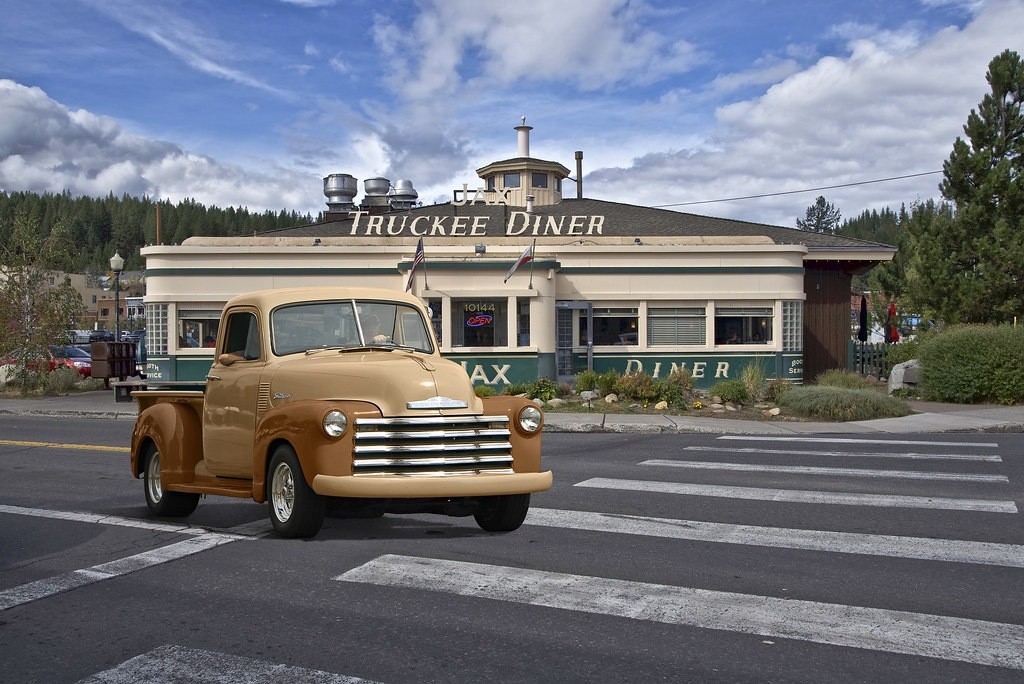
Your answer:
885,303,899,343
858,298,867,342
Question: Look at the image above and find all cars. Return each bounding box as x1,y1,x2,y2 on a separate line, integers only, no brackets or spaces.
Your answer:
89,329,115,342
121,327,146,342
0,344,92,380
55,328,93,344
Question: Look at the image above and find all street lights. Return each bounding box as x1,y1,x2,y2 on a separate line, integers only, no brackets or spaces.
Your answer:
109,250,125,342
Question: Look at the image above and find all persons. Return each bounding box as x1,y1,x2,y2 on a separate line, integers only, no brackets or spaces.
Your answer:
204,336,216,347
348,314,390,344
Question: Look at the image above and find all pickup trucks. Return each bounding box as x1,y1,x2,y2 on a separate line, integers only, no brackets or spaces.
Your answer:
130,286,552,540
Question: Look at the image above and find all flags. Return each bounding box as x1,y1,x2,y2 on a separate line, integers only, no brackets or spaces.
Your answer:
503,243,534,284
405,237,424,293
